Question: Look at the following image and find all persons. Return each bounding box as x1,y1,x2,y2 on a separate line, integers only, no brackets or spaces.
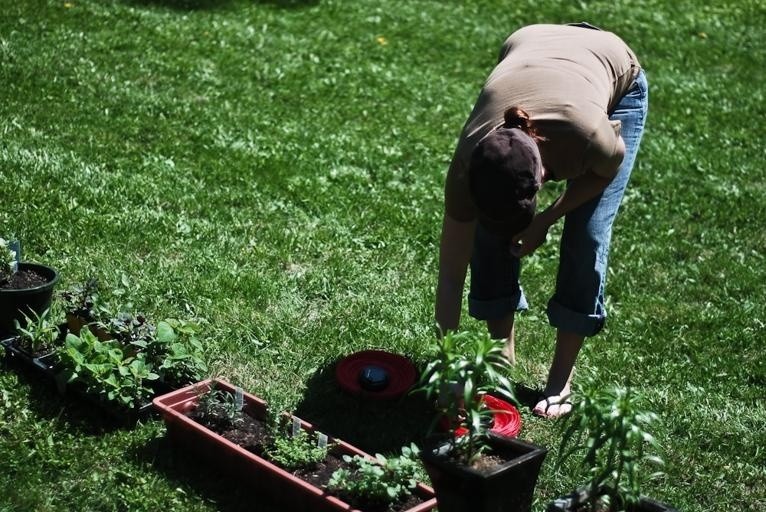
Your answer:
435,21,648,422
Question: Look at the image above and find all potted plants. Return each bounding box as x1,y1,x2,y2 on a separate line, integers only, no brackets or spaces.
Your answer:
394,318,550,509
1,238,59,335
153,375,438,510
542,377,677,510
2,271,201,427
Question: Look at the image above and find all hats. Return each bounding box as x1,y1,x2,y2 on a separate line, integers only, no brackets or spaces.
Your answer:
469,127,541,241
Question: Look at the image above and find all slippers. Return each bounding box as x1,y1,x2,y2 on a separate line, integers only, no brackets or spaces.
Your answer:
532,390,573,419
